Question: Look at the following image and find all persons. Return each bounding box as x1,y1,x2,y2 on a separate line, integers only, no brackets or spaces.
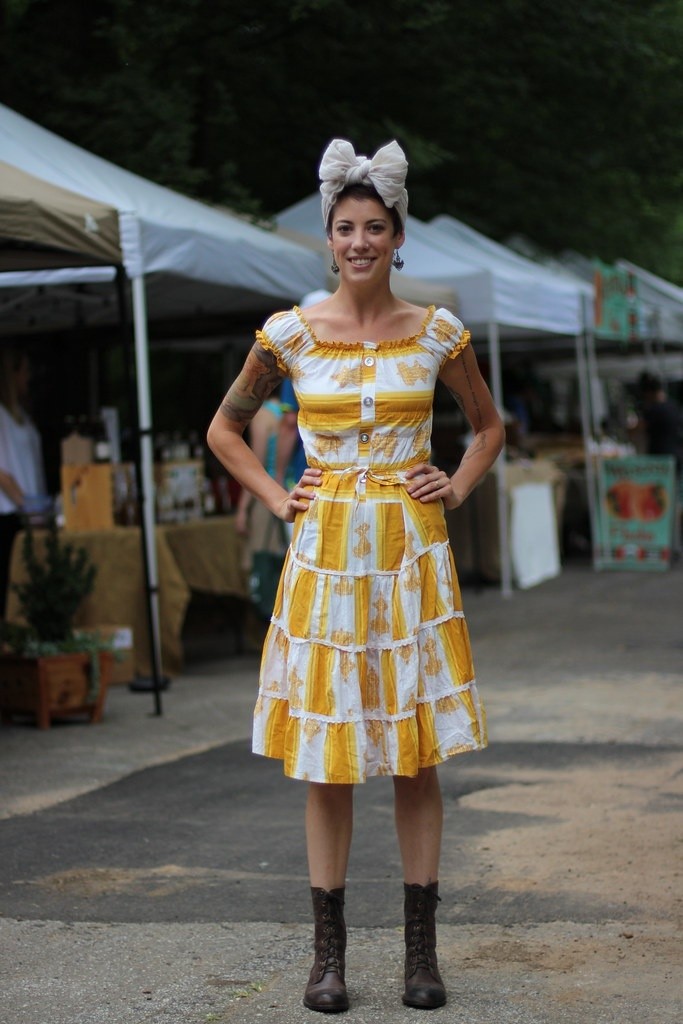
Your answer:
274,373,307,543
206,137,506,1013
0,348,50,620
234,348,296,650
639,368,683,556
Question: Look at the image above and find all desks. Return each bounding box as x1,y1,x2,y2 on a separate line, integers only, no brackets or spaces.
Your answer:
3,512,246,688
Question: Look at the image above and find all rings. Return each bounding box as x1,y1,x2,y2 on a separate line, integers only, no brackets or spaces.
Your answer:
436,480,439,489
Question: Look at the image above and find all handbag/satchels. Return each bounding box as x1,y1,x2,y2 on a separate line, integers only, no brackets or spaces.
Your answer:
248,514,290,618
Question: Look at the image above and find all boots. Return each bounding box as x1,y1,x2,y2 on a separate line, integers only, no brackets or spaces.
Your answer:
403,881,447,1009
303,886,349,1011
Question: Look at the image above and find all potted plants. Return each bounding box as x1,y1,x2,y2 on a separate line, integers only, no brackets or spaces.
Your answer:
1,496,113,734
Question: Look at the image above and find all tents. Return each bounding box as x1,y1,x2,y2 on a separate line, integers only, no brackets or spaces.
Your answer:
0,105,683,720
